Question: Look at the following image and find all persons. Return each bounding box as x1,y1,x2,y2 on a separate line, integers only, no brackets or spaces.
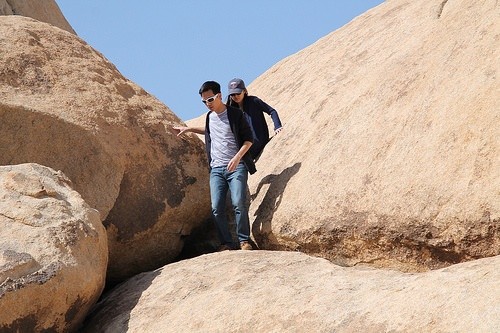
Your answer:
226,78,283,161
172,81,257,252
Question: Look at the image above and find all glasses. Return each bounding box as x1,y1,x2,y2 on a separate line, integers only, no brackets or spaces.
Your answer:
230,93,241,96
202,94,218,104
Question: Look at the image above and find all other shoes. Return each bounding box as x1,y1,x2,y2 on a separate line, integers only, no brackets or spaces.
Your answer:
217,243,234,252
240,241,252,250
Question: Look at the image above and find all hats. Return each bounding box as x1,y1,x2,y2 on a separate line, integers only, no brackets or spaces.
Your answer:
228,78,245,94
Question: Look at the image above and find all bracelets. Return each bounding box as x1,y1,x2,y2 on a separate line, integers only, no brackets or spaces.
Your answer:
236,153,242,159
